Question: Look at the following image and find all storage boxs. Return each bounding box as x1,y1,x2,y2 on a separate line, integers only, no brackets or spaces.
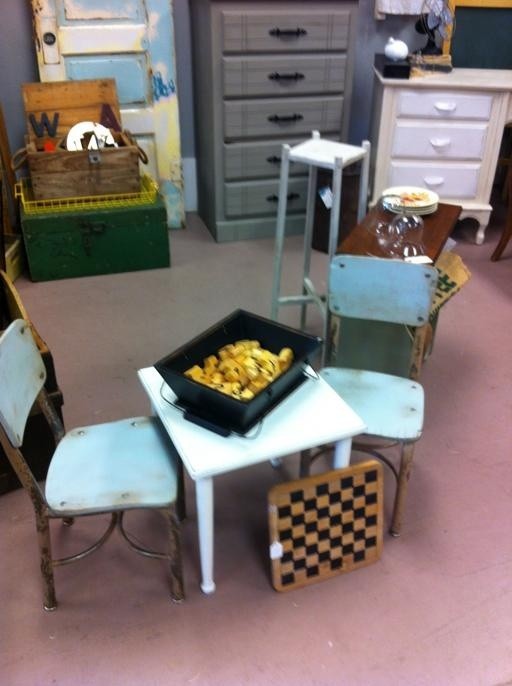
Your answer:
19,177,170,282
9,77,148,206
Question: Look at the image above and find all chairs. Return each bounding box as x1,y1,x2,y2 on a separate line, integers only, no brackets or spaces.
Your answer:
0,317,185,612
316,254,430,537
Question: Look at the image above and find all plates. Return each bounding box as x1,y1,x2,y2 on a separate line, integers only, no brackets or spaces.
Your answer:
380,183,439,216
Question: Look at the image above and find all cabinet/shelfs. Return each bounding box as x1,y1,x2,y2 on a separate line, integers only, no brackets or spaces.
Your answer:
367,63,512,245
189,0,359,246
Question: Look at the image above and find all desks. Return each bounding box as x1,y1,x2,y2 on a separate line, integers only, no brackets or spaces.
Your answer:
334,198,462,379
134,353,367,594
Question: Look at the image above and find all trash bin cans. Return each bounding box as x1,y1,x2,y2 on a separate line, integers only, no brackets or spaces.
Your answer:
311,167,361,254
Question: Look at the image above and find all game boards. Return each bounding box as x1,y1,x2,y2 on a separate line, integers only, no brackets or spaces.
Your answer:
269,460,385,592
